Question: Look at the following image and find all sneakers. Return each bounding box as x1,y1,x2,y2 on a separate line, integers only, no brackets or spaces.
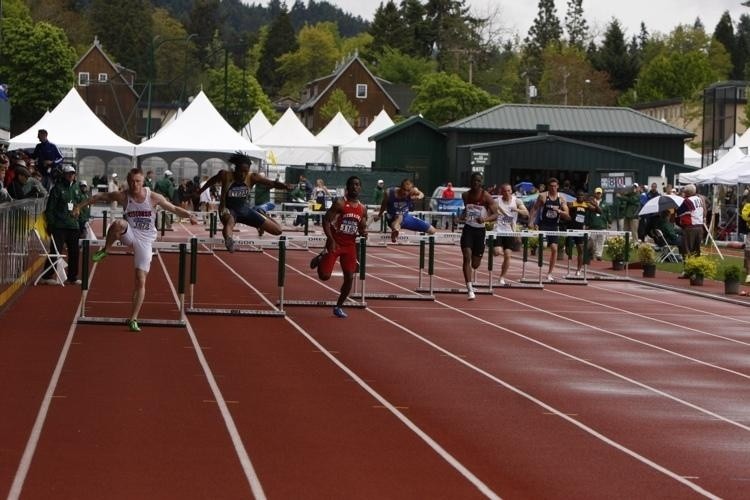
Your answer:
391,228,399,244
333,305,348,319
309,251,325,270
498,271,591,285
257,201,276,213
39,277,83,286
91,248,108,262
224,234,236,254
467,290,476,300
125,319,142,333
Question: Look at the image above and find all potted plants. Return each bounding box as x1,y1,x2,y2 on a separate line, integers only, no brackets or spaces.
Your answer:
514,223,744,294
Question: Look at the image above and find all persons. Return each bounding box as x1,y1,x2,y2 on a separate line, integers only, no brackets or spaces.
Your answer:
0,129,749,331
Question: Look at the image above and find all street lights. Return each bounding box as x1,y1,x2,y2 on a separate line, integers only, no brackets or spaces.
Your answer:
581,79,591,106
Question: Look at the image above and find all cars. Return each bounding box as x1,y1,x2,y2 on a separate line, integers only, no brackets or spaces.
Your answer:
425,181,578,218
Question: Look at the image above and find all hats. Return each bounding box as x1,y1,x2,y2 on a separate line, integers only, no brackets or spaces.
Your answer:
62,160,175,188
593,187,603,194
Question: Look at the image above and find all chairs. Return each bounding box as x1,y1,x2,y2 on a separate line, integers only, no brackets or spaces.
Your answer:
31,226,70,287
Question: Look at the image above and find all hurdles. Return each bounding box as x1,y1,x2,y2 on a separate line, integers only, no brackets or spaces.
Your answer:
160,210,215,254
383,211,424,245
186,237,290,318
77,238,192,328
107,201,335,234
414,209,458,246
350,229,632,300
103,209,162,256
361,203,388,248
213,210,264,252
259,209,311,251
276,235,370,308
304,209,329,248
